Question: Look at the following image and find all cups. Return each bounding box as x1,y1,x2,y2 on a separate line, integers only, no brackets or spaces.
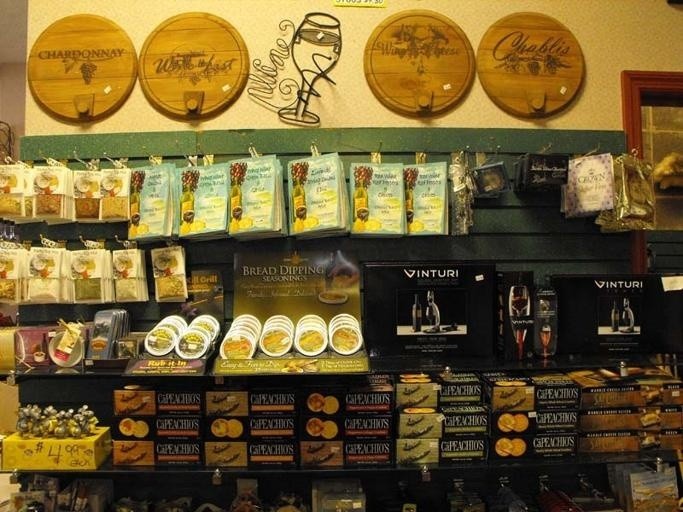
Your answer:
509,283,530,360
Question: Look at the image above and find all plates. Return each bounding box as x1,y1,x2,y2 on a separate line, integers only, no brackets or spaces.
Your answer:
145,315,188,356
260,315,294,357
294,315,328,357
49,332,84,368
87,358,129,369
220,314,261,360
329,314,363,356
319,291,348,304
175,314,220,360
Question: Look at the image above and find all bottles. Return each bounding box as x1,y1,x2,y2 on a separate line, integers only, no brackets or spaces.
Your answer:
130,184,140,226
42,333,48,359
413,294,423,332
405,182,414,211
230,177,242,221
292,176,306,222
182,184,195,224
354,180,369,221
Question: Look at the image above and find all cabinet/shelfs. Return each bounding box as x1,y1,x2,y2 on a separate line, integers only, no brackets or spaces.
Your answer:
0,125,682,512
